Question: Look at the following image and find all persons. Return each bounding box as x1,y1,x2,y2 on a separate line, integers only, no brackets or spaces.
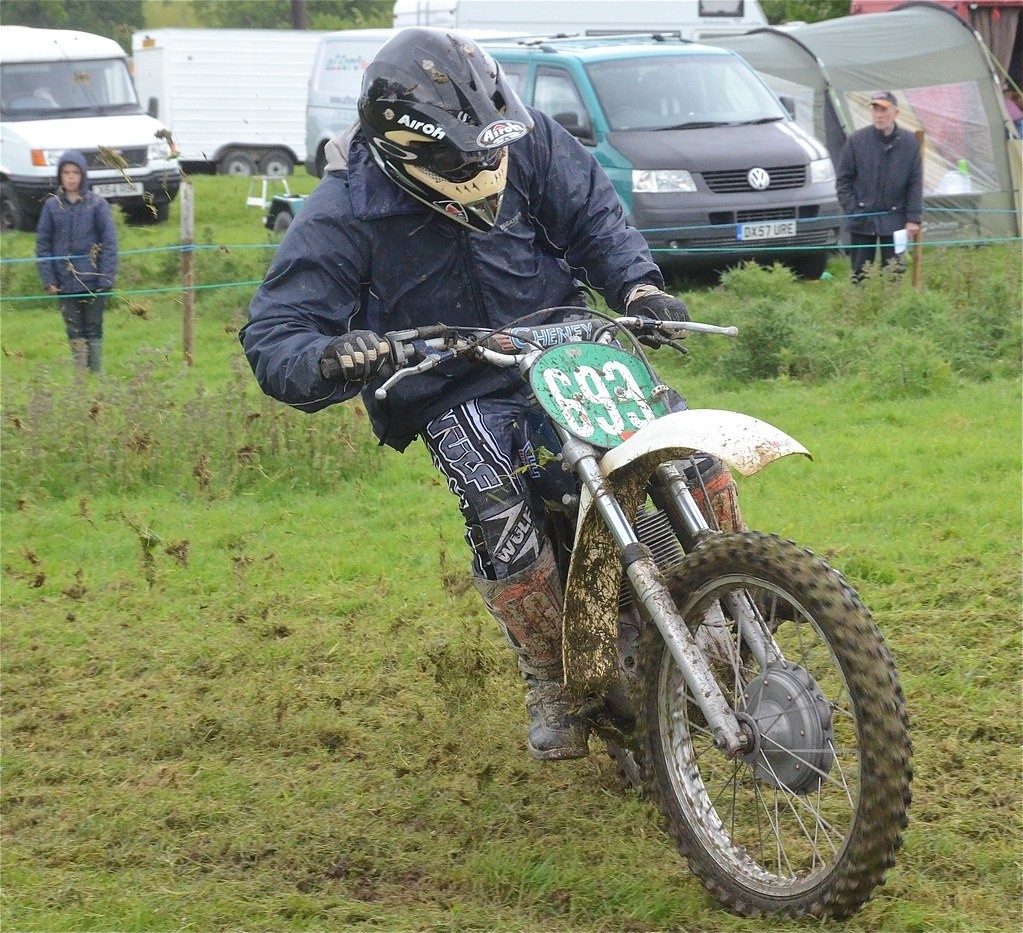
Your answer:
835,91,921,280
237,26,809,759
35,151,116,374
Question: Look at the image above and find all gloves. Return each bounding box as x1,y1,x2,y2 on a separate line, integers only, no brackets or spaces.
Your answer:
321,330,389,381
623,283,690,350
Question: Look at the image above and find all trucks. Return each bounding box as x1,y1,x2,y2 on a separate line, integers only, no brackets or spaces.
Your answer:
131,27,308,177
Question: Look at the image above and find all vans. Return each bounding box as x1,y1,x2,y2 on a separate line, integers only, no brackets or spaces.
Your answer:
304,27,533,181
0,25,183,233
482,32,843,280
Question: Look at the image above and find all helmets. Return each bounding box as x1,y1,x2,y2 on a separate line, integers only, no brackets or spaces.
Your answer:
356,25,535,233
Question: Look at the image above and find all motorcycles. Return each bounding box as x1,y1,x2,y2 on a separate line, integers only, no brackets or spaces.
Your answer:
322,308,916,928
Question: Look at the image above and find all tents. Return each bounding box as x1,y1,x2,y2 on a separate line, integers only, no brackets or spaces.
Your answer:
695,0,1022,247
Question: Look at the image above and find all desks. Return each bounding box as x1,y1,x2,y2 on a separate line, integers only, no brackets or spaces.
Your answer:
245,175,292,210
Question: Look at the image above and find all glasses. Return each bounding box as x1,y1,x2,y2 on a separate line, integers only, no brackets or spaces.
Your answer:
420,147,499,173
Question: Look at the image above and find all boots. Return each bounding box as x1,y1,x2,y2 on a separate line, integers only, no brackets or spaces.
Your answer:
471,534,590,760
685,459,810,622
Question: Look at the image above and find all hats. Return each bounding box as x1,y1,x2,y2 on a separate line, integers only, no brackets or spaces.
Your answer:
868,93,897,108
